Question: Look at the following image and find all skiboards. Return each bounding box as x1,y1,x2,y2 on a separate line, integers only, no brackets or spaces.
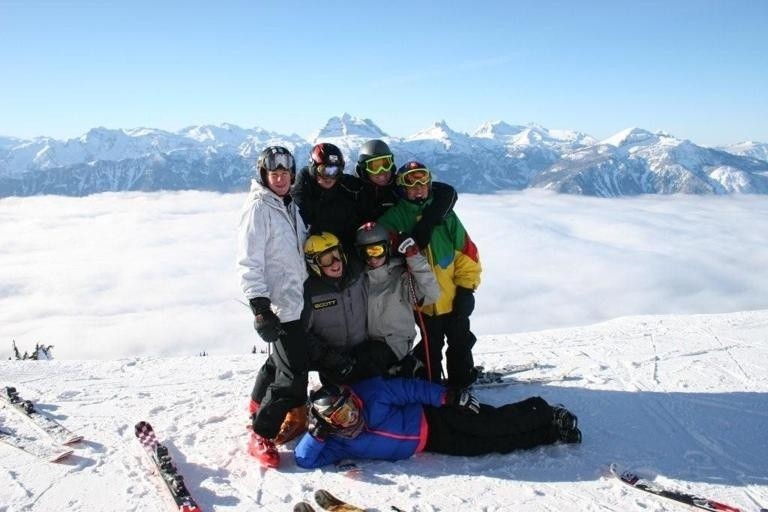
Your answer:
136,421,201,511
472,361,566,388
294,490,365,512
1,386,84,461
609,463,768,512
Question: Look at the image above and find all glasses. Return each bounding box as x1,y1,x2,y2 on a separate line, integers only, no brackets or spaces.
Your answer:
264,153,293,171
364,154,393,174
329,399,355,427
314,248,341,267
363,242,388,261
395,167,429,187
316,164,340,179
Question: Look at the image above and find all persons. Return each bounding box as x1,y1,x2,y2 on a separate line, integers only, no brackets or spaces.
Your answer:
288,377,586,469
239,141,311,469
272,139,481,445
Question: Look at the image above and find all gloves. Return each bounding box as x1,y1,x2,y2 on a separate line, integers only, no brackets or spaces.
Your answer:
307,391,327,438
446,389,480,416
392,230,418,258
451,285,474,321
323,342,352,371
411,208,440,251
249,297,285,342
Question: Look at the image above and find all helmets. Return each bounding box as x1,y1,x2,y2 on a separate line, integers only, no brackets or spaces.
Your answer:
255,145,295,184
303,231,347,277
396,161,425,178
311,383,350,425
355,223,388,248
310,143,342,165
357,139,391,163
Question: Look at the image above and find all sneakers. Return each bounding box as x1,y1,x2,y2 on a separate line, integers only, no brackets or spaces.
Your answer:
556,425,581,444
551,406,577,432
247,398,307,468
